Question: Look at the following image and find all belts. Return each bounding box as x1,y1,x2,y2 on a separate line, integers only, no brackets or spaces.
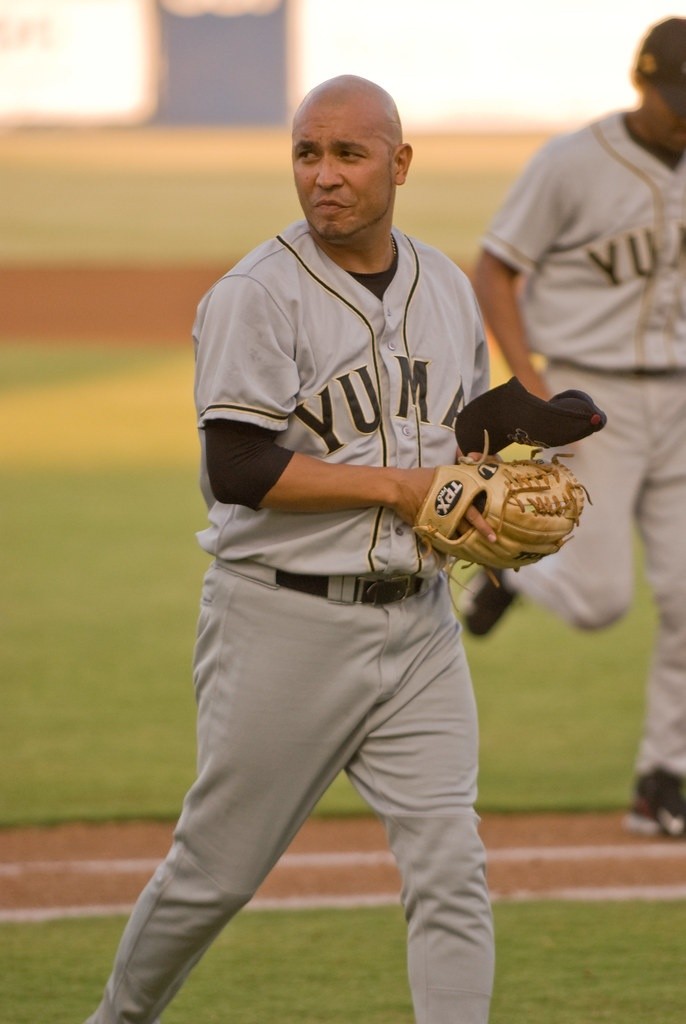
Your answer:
275,568,424,606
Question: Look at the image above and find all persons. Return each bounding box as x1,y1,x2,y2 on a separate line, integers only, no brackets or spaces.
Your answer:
82,75,587,1022
455,17,685,840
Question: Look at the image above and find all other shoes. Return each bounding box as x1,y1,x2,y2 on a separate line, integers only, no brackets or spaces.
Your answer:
458,563,517,636
625,766,686,840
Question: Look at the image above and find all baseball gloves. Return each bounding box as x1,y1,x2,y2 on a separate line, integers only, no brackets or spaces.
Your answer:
413,450,588,573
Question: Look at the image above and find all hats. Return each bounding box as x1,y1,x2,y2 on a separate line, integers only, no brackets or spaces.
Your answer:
637,17,686,91
454,375,607,456
